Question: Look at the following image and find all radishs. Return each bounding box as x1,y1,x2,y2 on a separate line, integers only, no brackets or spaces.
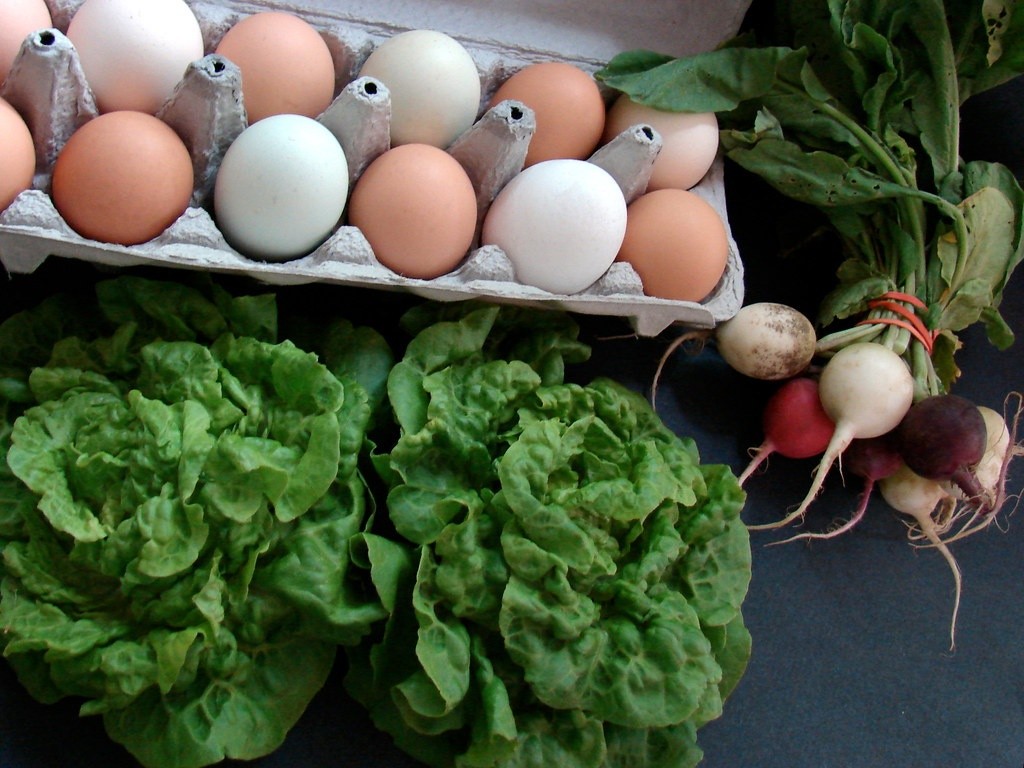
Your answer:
648,301,815,414
879,405,1012,647
741,342,913,527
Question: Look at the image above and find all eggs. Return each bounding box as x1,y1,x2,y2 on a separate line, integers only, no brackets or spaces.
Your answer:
214,113,349,263
214,11,335,126
482,158,628,297
357,29,481,150
487,61,606,169
598,91,720,190
0,0,210,244
616,189,728,303
350,143,478,279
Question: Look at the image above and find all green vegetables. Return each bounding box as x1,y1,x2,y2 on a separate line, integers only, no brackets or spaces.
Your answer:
0,268,753,768
